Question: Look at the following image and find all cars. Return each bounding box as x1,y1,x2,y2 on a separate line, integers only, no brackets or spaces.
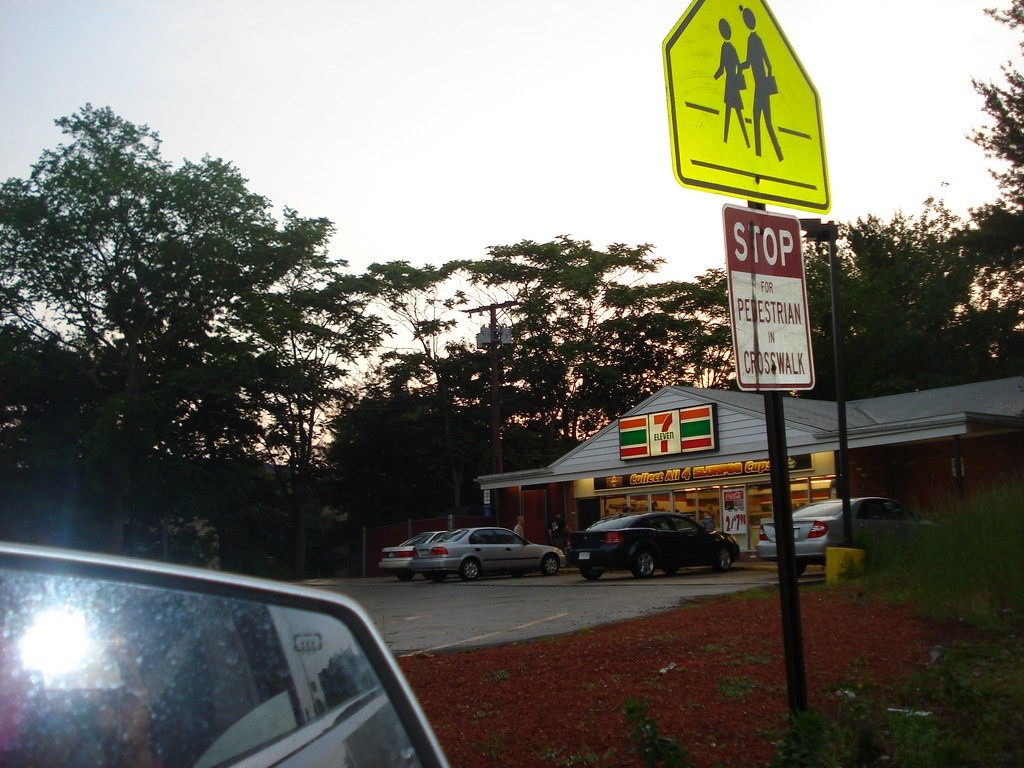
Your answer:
378,530,486,581
408,527,567,582
563,510,740,581
756,496,935,578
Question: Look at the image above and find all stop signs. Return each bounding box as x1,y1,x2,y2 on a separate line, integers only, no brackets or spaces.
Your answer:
725,208,807,280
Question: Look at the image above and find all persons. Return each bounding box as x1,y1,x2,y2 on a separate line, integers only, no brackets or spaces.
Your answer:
548,510,568,555
7,626,162,767
514,515,526,544
702,511,714,529
